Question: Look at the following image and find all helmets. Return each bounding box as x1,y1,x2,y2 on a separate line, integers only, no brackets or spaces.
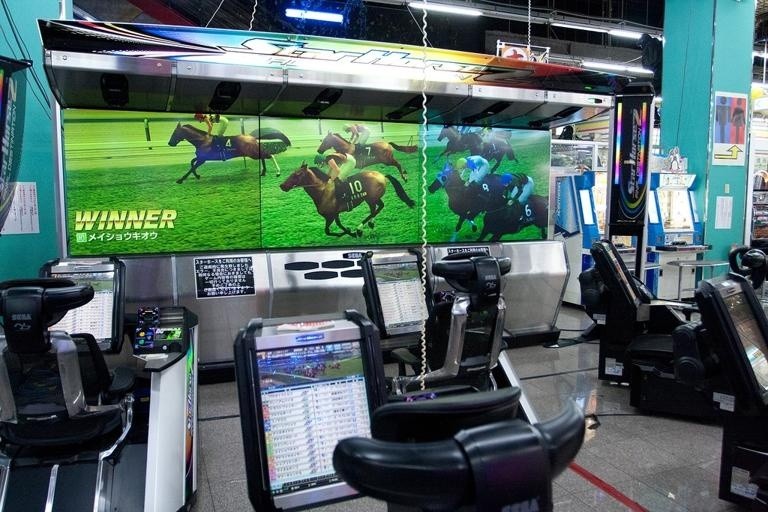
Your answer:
456,157,467,169
342,123,351,131
312,154,326,164
193,113,203,119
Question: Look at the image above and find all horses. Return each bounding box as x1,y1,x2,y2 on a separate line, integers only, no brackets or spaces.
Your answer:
167,121,291,184
317,129,418,183
427,125,549,242
279,159,417,238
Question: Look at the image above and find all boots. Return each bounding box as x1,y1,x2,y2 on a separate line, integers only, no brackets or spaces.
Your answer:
345,200,354,212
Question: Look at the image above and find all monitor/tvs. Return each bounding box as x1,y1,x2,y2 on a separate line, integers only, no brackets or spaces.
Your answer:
39,256,126,355
232,310,387,512
695,273,768,368
592,186,607,234
590,239,641,309
361,247,435,337
658,190,691,230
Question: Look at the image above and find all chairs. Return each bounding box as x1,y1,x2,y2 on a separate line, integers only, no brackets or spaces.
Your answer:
0,274,138,461
576,236,767,512
334,236,586,512
